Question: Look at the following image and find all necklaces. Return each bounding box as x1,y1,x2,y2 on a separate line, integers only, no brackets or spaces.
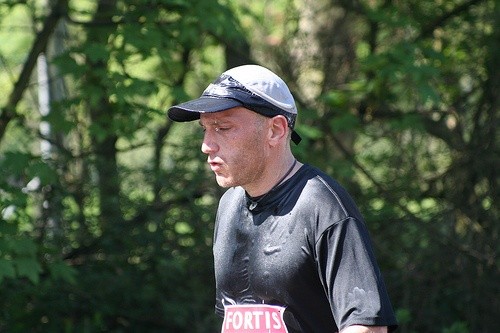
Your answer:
245,160,296,210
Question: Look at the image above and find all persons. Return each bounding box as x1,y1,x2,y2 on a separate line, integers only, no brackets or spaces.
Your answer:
167,64,398,333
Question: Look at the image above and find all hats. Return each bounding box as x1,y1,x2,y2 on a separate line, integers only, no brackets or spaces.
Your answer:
168,64,302,145
